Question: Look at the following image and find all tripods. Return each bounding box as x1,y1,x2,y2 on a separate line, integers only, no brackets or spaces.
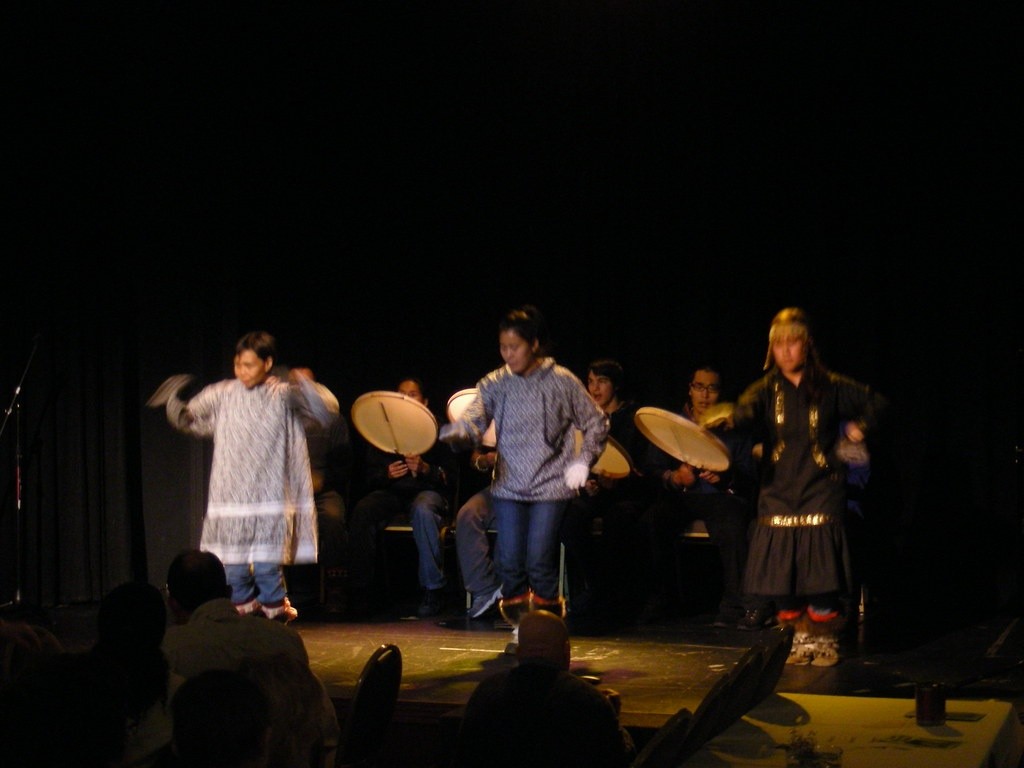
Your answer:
0,333,78,612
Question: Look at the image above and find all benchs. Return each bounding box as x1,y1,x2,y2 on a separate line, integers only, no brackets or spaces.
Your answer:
318,509,852,608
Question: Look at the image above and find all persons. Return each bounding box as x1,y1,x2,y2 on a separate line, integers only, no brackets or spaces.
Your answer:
162,306,871,668
0,550,633,768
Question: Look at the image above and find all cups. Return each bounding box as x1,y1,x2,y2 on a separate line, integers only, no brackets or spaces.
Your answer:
915,682,946,725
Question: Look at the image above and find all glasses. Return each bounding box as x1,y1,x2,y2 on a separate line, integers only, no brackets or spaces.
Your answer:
688,382,721,394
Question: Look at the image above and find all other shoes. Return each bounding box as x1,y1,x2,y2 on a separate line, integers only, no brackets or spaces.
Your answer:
736,601,777,631
810,627,840,668
468,583,504,618
712,598,743,628
417,576,456,620
785,632,815,666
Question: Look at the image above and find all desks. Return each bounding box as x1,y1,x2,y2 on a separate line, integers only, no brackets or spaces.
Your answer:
682,693,1024,768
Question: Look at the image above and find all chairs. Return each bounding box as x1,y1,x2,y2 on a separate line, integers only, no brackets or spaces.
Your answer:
335,644,402,768
631,625,795,768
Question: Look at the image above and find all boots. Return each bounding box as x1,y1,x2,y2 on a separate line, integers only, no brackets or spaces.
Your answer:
236,599,261,618
262,596,298,626
499,593,531,627
528,589,567,622
